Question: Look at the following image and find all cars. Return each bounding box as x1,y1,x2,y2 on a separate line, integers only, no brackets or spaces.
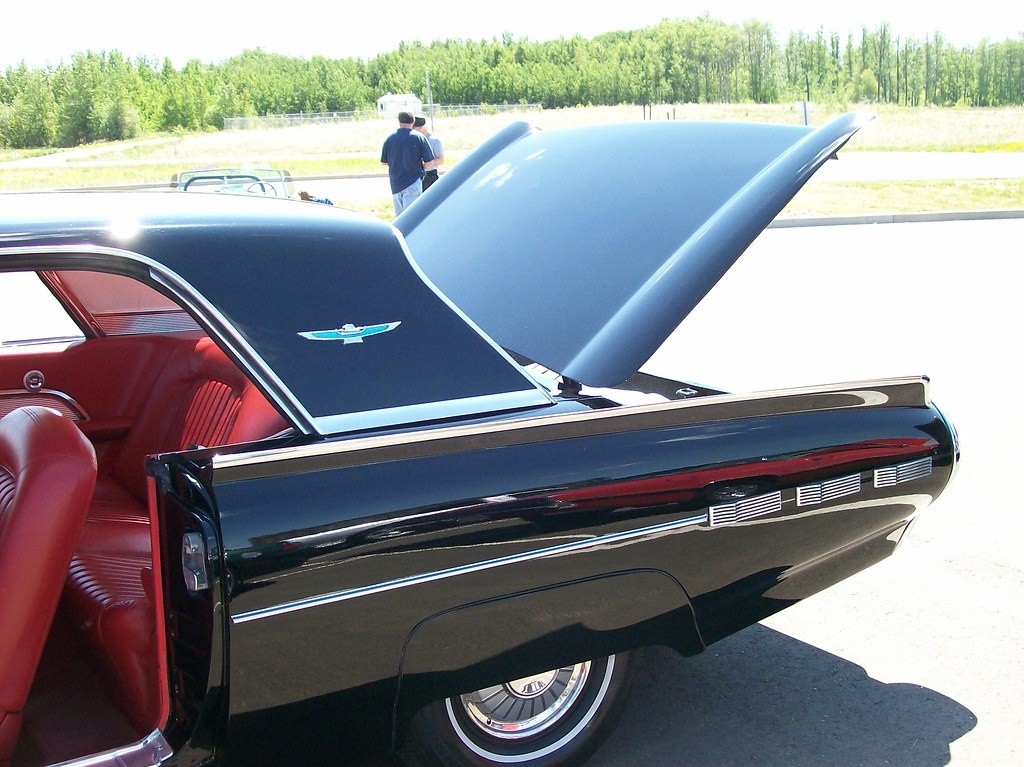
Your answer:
0,108,964,767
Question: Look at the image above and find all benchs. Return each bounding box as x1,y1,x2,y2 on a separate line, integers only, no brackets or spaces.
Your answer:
65,335,288,730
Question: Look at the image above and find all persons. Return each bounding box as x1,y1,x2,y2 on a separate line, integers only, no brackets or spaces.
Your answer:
380,111,435,217
412,114,445,194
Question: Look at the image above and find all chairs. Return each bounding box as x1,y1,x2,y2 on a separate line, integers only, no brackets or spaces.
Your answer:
0,407,99,767
